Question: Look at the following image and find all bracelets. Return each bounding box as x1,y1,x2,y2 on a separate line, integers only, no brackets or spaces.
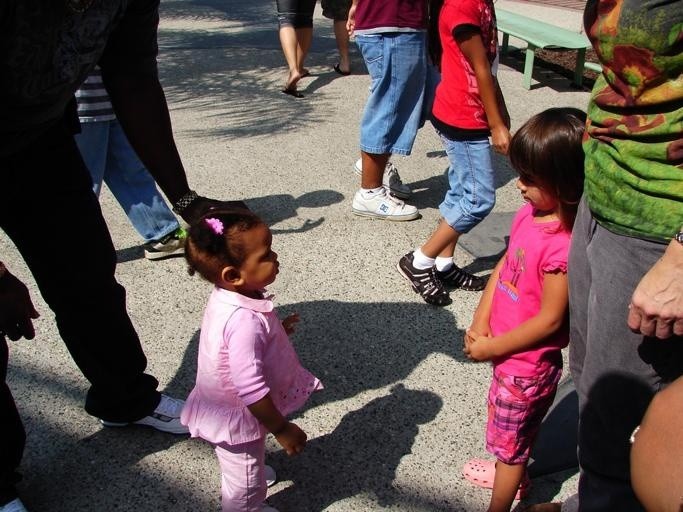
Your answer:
672,231,682,244
172,191,198,214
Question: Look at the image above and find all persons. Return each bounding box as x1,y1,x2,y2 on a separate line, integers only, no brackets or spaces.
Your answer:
461,106,585,510
398,1,512,305
567,0,683,509
75,64,191,261
630,374,682,511
179,207,323,510
345,0,441,220
276,0,317,98
321,0,353,75
1,0,249,511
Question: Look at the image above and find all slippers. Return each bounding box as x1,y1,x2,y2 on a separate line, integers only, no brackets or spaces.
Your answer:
462,457,529,499
282,86,304,98
334,63,350,75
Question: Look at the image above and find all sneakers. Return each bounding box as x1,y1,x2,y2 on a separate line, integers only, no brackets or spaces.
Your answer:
351,184,419,220
434,263,486,292
354,158,411,199
396,251,452,306
145,228,188,260
265,463,277,486
99,393,190,434
0,498,28,511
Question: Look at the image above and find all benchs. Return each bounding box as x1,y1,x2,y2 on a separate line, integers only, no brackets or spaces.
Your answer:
494,7,593,91
